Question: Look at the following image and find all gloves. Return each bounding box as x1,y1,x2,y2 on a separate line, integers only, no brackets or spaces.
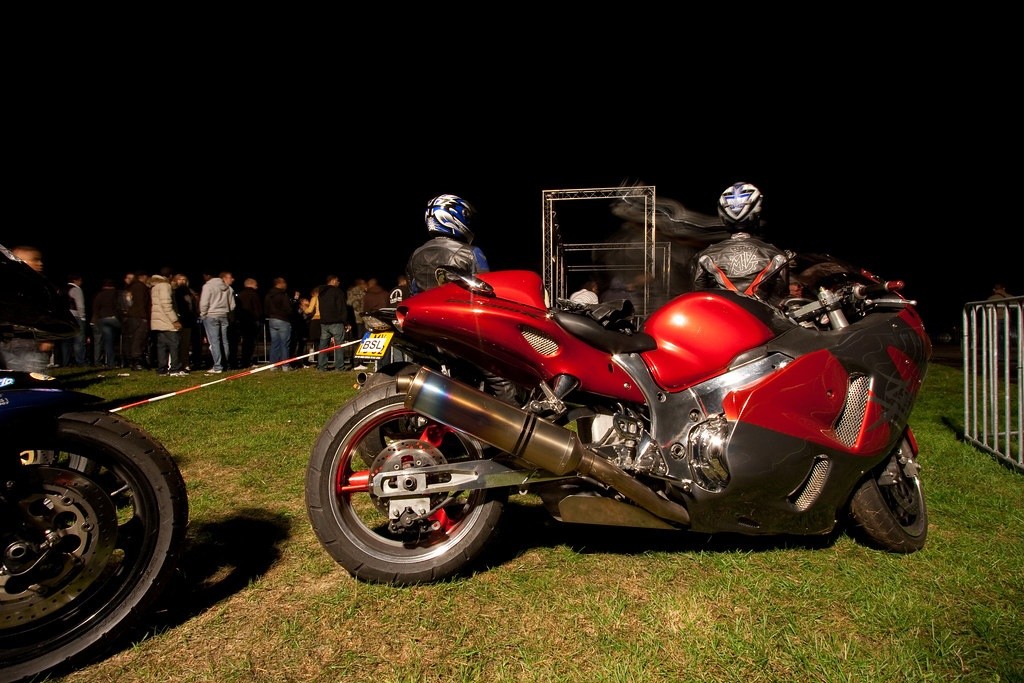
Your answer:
556,296,636,336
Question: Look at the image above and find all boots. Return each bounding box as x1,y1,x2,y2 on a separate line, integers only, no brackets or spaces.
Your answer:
131,352,152,371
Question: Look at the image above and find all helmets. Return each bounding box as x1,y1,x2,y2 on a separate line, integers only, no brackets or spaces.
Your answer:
718,182,764,231
425,194,478,245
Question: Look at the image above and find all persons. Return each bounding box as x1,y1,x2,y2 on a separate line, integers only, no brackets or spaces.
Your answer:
599,276,632,304
59,267,414,377
985,282,1017,353
570,281,599,304
0,245,55,464
406,194,519,410
693,181,789,308
784,250,804,297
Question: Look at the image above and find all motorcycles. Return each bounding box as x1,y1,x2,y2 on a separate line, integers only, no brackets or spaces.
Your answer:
352,286,650,469
301,246,930,589
0,238,192,683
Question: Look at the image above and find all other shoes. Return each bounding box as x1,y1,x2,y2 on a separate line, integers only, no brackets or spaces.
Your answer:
282,366,290,371
335,366,352,371
159,369,169,376
313,365,328,371
207,368,222,373
170,369,191,377
250,364,258,367
270,367,281,371
297,364,310,369
